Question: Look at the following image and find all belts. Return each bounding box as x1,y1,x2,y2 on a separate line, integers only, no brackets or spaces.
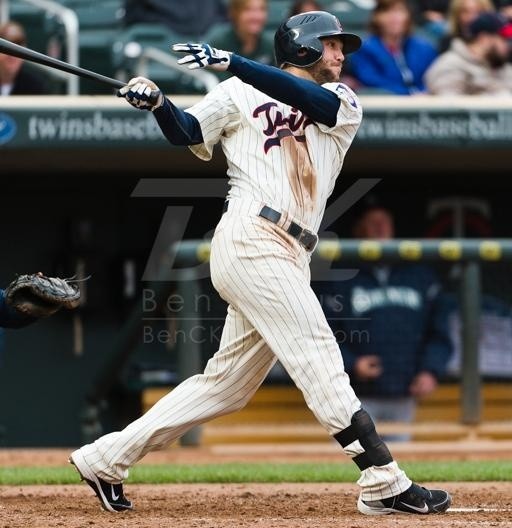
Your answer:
220,201,318,253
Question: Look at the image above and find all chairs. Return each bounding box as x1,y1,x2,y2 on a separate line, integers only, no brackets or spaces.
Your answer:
1,2,376,92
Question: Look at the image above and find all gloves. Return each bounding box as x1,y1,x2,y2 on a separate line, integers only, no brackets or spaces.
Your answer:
172,42,230,71
117,77,164,111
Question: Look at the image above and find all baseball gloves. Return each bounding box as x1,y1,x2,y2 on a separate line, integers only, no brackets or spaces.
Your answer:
5,272,80,320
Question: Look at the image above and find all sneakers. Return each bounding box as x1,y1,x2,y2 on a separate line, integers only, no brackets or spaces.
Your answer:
356,481,450,517
70,455,132,513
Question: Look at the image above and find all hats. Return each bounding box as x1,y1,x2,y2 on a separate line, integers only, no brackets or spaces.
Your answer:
469,12,512,39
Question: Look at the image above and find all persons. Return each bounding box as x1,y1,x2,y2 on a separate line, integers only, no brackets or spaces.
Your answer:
68,10,454,518
320,198,458,441
0,22,69,97
0,270,95,330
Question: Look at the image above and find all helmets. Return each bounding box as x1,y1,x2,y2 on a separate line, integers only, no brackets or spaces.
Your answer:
274,10,362,72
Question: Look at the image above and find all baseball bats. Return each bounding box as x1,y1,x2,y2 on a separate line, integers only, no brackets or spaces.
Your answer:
0,37,160,105
